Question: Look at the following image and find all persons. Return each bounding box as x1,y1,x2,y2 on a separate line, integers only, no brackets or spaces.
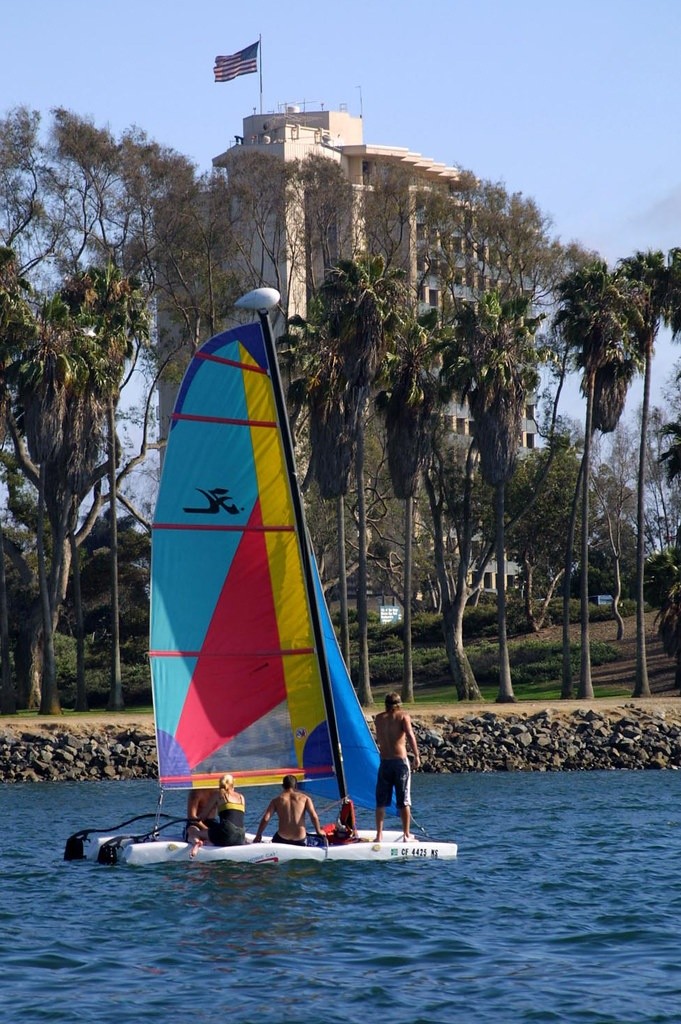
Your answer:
374,692,420,842
182,774,246,860
253,775,326,846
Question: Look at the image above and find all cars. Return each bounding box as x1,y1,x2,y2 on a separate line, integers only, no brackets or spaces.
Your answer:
588,594,613,607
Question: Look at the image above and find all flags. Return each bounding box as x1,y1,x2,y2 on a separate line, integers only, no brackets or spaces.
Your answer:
214,41,259,83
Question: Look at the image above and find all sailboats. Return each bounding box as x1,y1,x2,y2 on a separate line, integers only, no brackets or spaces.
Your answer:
59,285,459,865
